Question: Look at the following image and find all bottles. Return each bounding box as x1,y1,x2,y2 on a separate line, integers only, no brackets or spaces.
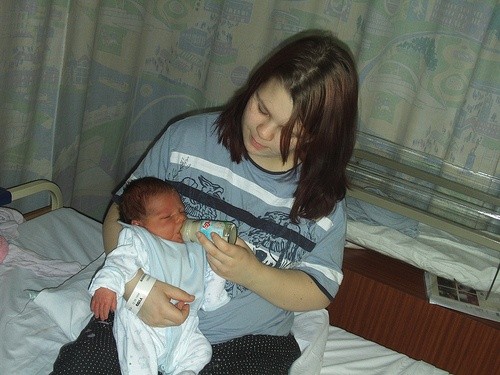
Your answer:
180,218,238,247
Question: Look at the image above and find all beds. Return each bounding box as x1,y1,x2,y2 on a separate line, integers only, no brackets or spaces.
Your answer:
0,178,453,375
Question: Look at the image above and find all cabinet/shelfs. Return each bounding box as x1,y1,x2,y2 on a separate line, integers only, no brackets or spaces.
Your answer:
327,247,500,375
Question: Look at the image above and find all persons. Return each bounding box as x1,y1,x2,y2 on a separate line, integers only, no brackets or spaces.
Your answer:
88,176,231,375
50,30,359,375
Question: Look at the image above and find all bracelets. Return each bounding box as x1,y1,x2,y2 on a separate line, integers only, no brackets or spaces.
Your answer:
125,273,157,315
